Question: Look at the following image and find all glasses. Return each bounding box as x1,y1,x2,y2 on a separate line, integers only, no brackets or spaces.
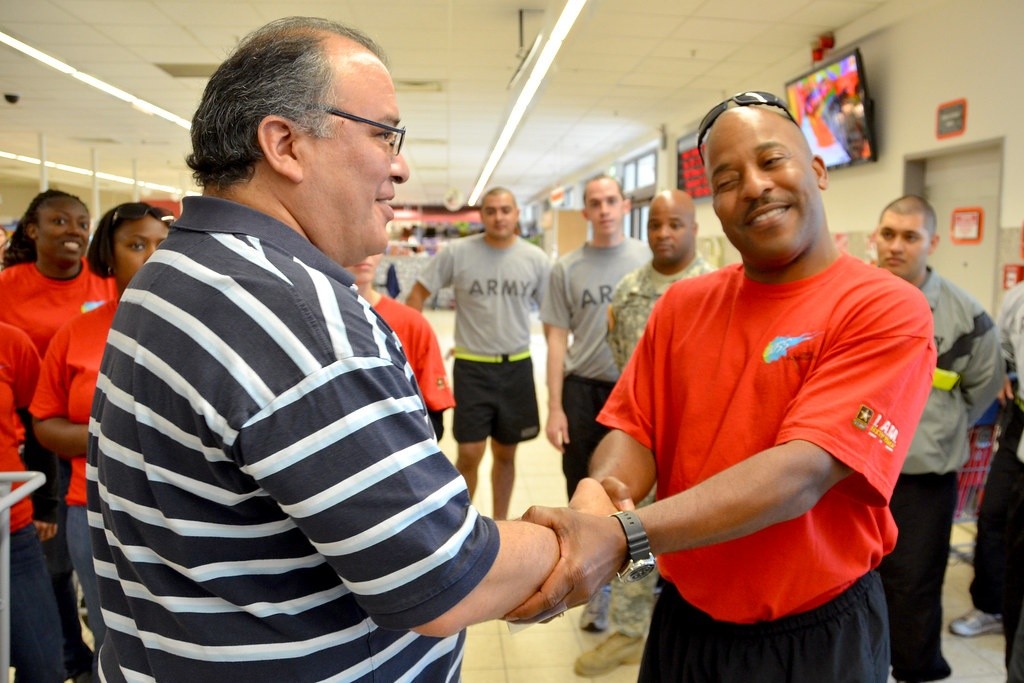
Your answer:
302,103,405,156
697,91,799,166
112,202,176,228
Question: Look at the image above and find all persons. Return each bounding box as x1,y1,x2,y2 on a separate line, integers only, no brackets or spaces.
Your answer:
407,189,555,519
0,190,93,683
347,252,457,445
503,92,938,683
85,16,616,683
30,201,178,656
537,173,653,637
573,190,720,676
951,279,1024,637
874,194,1006,683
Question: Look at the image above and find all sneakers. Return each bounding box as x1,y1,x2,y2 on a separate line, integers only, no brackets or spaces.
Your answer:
582,585,616,632
949,606,1004,636
574,629,645,676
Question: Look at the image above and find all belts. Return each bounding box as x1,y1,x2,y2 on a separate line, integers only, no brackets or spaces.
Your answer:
454,349,531,363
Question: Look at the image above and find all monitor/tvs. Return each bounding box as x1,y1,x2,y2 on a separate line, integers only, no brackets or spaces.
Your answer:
786,49,877,171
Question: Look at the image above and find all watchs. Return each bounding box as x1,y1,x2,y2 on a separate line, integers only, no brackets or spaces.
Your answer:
607,510,657,583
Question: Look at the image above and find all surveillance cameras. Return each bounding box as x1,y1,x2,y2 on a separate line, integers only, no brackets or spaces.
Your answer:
5,94,18,104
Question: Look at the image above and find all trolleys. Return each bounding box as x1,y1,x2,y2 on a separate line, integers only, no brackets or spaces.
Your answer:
948,369,1019,565
